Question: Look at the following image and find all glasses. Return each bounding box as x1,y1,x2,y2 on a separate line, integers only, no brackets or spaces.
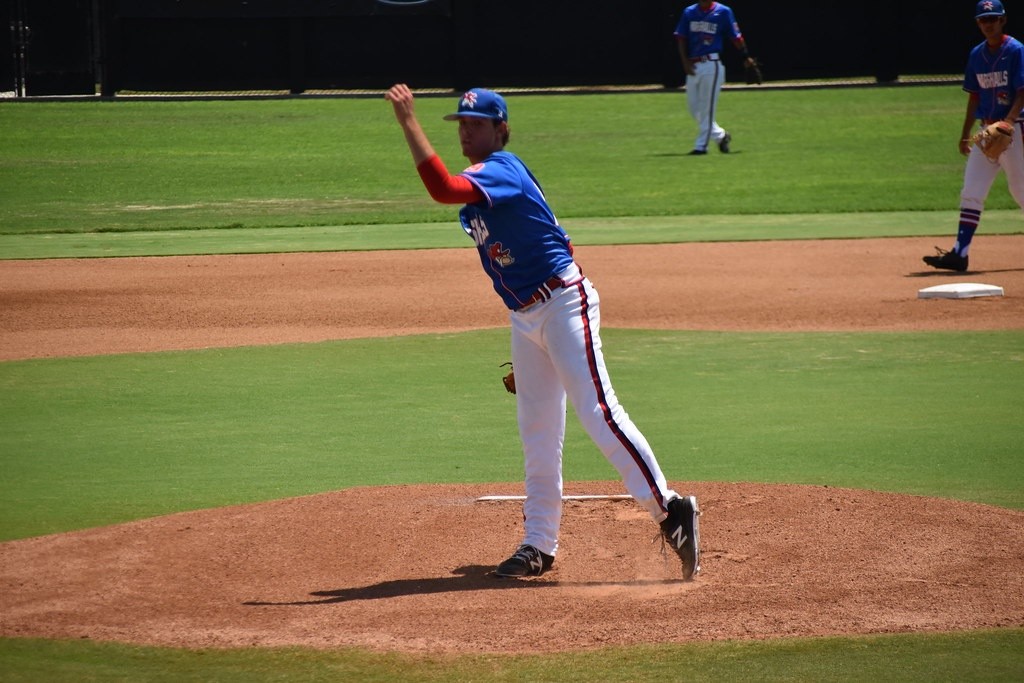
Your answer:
980,17,1000,24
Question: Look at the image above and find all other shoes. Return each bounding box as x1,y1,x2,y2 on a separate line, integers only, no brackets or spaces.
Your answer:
689,149,706,155
719,131,730,153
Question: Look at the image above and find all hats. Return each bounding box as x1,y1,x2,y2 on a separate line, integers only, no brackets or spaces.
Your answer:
443,87,508,122
975,0,1005,18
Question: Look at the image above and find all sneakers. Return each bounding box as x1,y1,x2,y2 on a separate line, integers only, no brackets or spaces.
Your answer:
650,496,703,580
922,247,969,272
495,545,555,577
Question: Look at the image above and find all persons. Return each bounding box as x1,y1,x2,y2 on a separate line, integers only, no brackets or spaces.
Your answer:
674,0,762,156
922,0,1024,272
385,83,700,582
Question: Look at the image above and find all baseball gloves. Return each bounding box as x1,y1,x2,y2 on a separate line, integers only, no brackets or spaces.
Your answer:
744,57,762,85
962,117,1016,165
497,360,517,396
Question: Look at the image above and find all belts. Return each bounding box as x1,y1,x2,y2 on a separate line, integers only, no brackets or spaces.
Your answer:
691,55,710,63
519,262,582,310
982,118,1002,124
